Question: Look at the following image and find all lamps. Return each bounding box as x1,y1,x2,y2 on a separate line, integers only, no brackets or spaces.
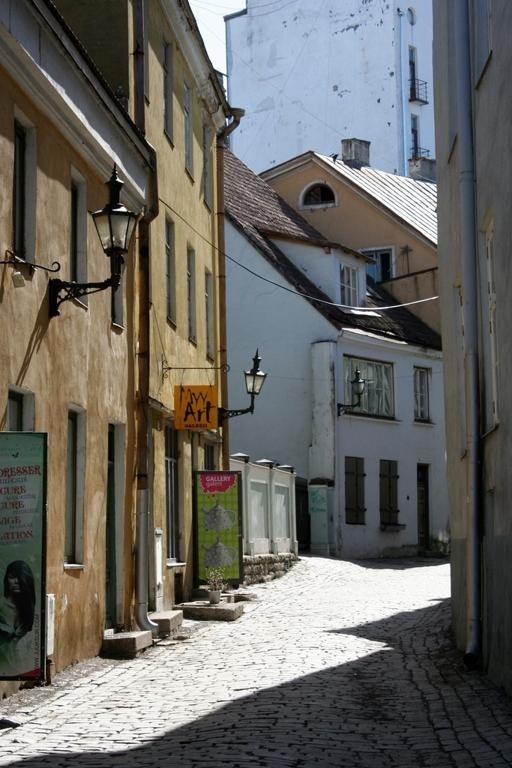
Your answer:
216,347,267,429
49,160,144,323
337,366,366,418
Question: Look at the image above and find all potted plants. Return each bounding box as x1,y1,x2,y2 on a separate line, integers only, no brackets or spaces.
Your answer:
206,567,225,606
